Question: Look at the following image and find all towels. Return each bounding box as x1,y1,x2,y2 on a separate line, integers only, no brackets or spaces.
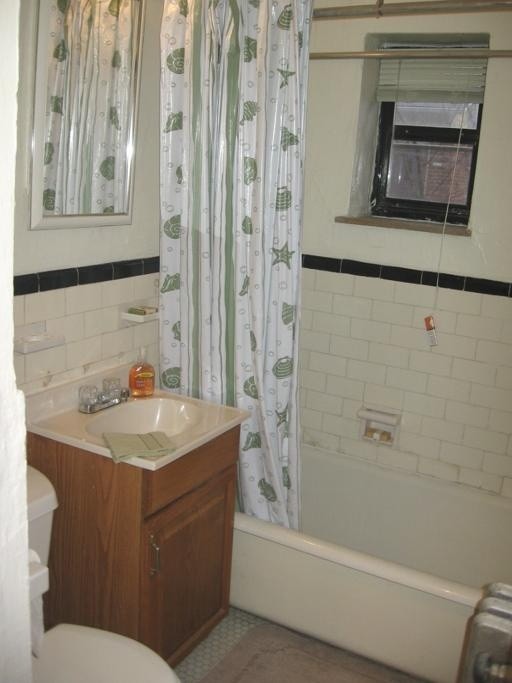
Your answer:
102,432,178,464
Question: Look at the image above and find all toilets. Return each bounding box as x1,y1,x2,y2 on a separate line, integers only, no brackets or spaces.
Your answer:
25,466,179,682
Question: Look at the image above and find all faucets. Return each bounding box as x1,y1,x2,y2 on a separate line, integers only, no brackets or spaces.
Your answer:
99,389,130,404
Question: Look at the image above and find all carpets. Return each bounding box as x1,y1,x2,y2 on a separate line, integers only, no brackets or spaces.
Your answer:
196,623,426,682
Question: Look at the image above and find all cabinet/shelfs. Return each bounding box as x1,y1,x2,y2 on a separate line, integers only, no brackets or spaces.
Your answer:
27,425,240,671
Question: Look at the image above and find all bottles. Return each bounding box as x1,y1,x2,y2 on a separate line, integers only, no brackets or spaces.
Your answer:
127,343,156,399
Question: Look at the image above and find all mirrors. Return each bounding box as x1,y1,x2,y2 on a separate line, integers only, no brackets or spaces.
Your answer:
30,1,145,228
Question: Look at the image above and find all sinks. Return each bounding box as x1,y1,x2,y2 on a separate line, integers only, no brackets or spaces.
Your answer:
84,399,201,439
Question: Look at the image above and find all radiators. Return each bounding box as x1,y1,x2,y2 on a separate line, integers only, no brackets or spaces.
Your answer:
457,583,512,682
